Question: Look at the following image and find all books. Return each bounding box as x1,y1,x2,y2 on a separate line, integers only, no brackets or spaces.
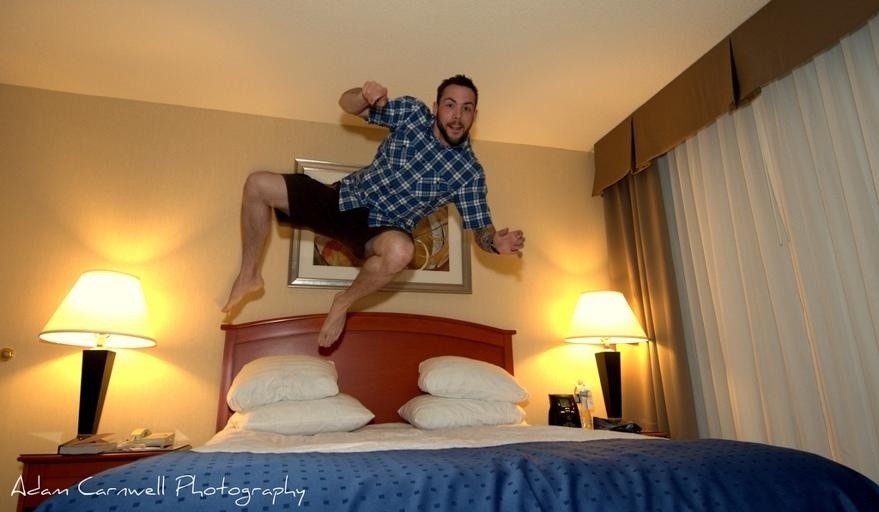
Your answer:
57,432,121,454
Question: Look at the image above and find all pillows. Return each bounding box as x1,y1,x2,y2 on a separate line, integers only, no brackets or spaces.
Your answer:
394,352,530,432
223,351,377,437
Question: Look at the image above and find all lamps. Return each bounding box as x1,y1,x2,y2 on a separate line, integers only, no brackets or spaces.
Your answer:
558,288,653,432
36,267,160,459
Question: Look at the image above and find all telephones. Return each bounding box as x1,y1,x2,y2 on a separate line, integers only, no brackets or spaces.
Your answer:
130,428,176,449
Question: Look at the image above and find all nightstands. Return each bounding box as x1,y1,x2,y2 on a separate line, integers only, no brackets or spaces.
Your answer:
12,444,172,510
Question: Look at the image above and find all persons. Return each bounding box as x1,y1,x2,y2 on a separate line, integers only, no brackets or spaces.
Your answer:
220,74,526,348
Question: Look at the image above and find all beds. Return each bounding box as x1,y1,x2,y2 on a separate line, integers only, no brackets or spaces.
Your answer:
28,309,879,511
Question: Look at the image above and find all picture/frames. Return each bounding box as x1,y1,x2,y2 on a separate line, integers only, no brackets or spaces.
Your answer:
284,155,475,297
